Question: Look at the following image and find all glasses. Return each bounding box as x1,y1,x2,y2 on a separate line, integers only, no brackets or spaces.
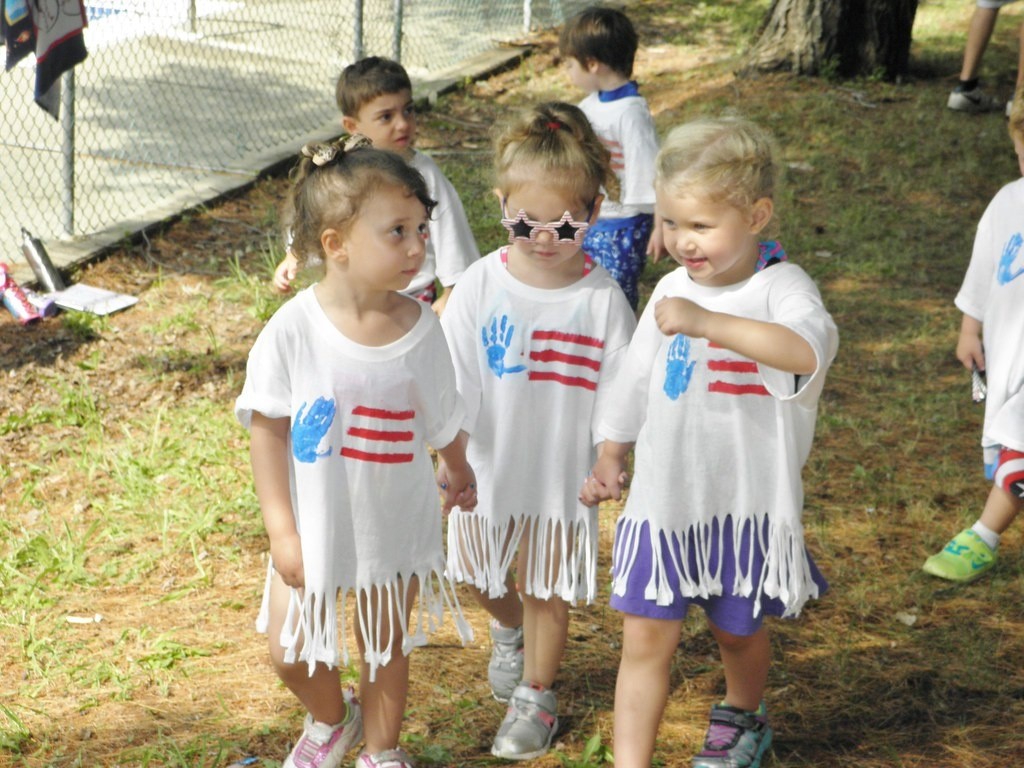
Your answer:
501,200,592,245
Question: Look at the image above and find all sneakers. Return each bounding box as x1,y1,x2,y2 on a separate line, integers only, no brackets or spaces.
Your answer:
923,525,1005,584
492,678,559,759
357,747,411,768
282,688,361,768
946,86,1016,118
488,620,525,703
689,702,774,768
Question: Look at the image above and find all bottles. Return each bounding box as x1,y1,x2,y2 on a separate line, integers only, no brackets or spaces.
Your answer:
20,227,65,294
19,284,57,318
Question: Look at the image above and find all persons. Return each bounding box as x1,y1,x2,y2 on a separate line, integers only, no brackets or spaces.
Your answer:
947,0,1024,116
923,85,1024,583
592,115,839,768
434,103,638,761
273,57,482,317
233,134,477,768
557,7,658,315
